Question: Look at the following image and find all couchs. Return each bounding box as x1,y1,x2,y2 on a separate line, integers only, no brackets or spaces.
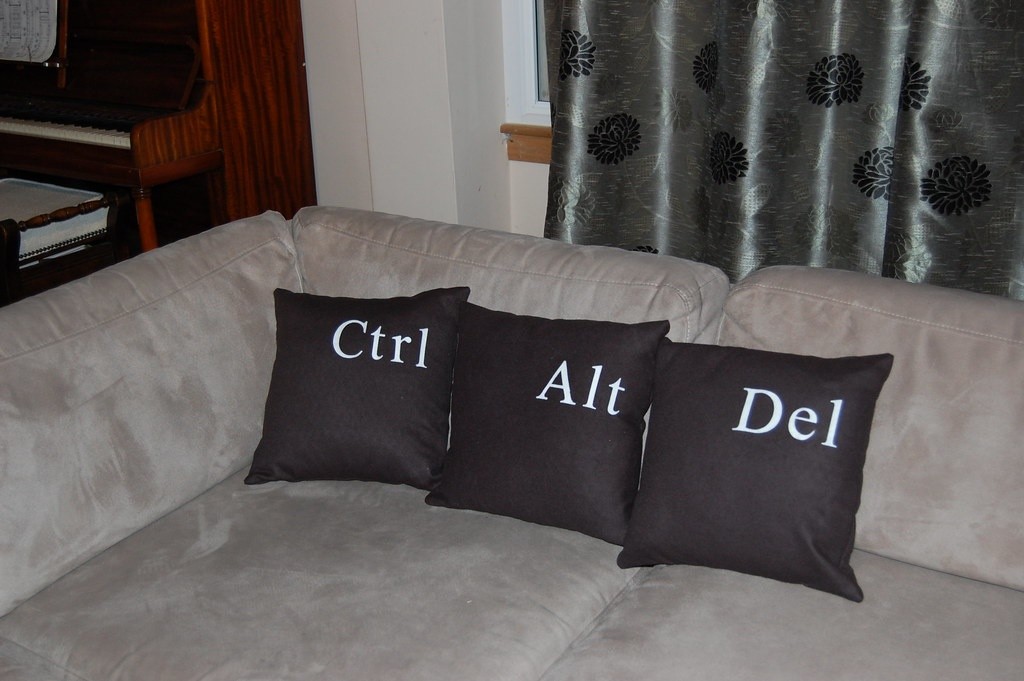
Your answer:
0,204,1024,681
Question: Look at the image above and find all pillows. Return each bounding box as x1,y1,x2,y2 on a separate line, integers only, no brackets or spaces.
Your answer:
425,302,669,543
244,284,472,488
618,337,894,601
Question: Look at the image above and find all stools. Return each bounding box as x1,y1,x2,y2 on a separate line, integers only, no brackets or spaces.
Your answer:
0,178,119,307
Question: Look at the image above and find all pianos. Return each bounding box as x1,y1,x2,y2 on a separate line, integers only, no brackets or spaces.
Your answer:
0,0,318,253
